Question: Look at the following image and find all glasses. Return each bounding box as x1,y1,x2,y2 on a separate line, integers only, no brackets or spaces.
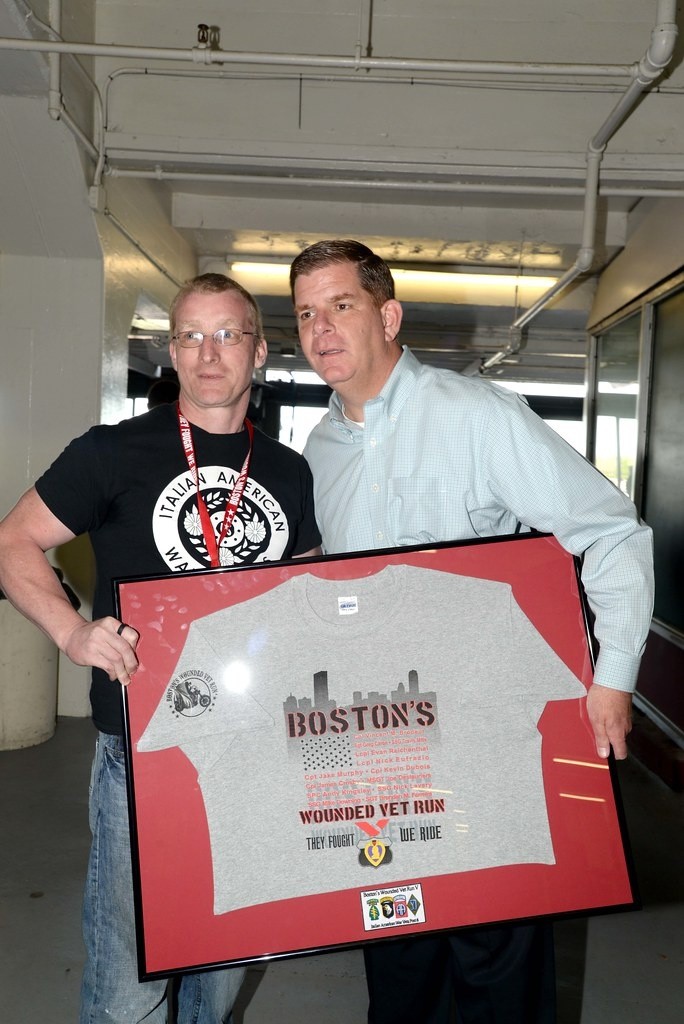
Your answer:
171,328,259,346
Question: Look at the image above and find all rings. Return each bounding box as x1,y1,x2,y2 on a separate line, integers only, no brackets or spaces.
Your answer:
117,623,126,635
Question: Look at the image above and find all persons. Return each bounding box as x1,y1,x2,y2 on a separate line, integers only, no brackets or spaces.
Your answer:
287,238,657,1024
0,272,322,1024
